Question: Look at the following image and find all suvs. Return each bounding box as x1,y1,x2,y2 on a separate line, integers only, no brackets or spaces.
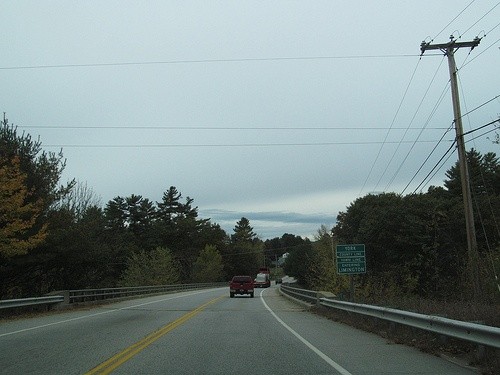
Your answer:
230,275,254,298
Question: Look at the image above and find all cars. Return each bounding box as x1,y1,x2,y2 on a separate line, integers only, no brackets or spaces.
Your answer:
254,277,270,288
276,278,283,285
